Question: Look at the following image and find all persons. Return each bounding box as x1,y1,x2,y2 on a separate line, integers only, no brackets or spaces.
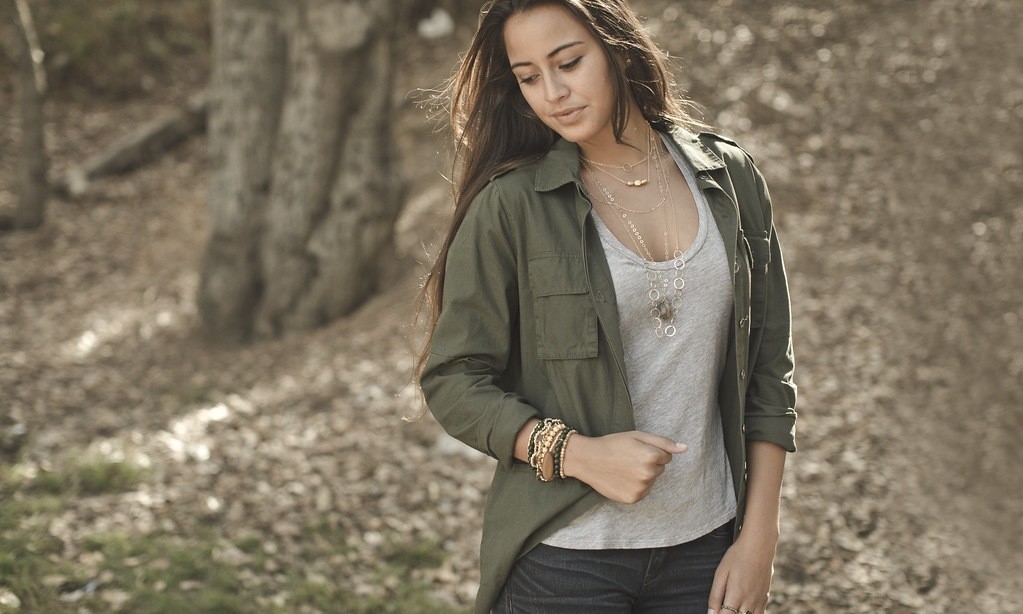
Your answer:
417,2,799,613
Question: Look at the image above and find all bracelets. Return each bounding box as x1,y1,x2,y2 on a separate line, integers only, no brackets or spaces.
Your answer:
527,415,579,484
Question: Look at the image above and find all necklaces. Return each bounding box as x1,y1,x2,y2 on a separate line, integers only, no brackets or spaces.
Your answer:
573,120,686,340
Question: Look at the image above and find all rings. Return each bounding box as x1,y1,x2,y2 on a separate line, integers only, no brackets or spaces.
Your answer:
721,604,740,614
741,610,752,614
